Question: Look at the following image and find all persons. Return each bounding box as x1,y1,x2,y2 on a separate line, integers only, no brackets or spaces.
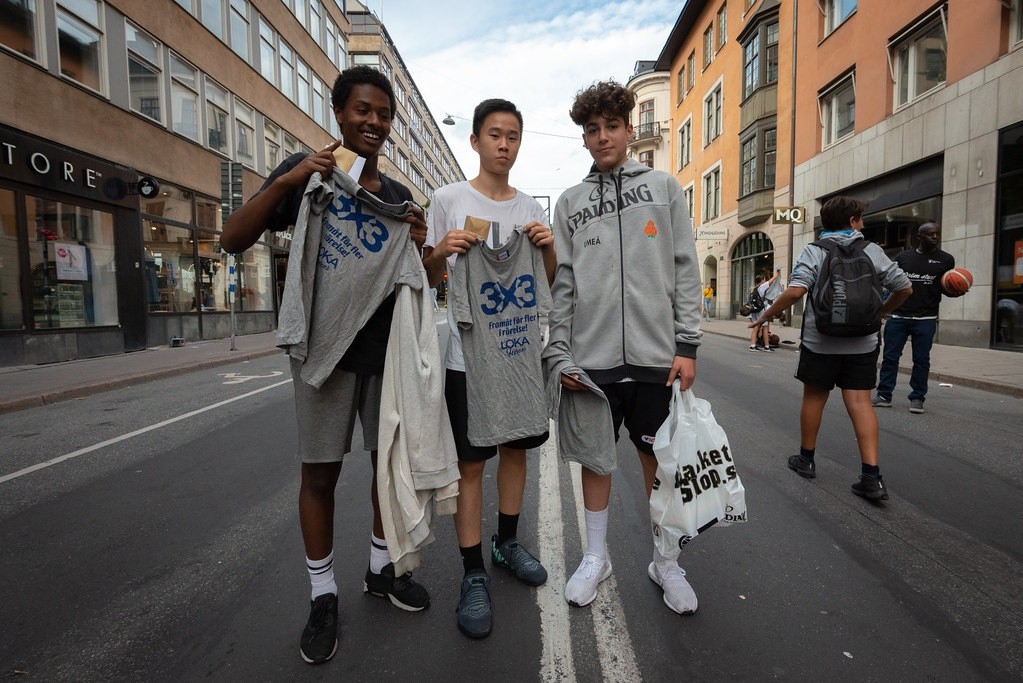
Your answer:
873,223,969,415
748,197,913,502
554,82,704,615
423,98,558,639
749,271,776,352
703,283,714,322
220,66,428,663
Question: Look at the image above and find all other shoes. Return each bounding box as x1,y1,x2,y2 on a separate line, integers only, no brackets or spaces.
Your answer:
749,346,761,352
764,345,774,352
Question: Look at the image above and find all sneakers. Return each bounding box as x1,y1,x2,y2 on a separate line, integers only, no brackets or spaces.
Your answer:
455,570,492,639
564,552,612,608
647,561,698,616
870,396,892,408
363,559,432,611
490,534,548,587
909,399,925,413
300,593,340,663
788,454,815,478
850,474,889,501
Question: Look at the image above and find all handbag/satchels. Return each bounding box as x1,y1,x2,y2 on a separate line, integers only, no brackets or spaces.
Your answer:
703,304,707,315
740,305,751,317
651,378,748,560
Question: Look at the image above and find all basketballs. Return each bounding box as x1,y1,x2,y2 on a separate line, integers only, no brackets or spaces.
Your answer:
941,268,974,296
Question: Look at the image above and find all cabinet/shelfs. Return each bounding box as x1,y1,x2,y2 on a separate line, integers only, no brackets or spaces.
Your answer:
57,283,87,328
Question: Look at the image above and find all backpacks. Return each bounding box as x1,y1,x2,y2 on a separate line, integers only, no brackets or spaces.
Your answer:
807,238,884,338
747,285,770,313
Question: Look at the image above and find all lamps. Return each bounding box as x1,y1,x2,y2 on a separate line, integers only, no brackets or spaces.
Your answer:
442,115,455,125
